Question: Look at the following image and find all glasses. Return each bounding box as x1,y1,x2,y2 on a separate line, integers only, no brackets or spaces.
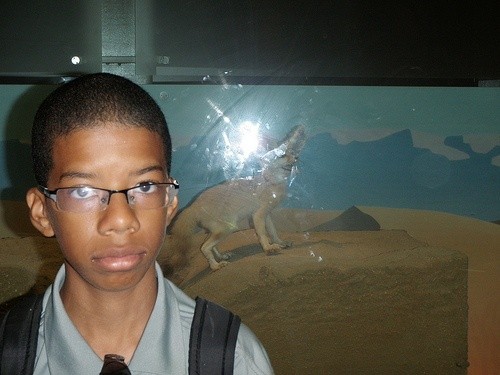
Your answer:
36,177,180,213
98,353,132,375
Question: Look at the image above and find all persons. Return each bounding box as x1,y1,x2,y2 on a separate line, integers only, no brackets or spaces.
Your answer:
1,72,274,375
